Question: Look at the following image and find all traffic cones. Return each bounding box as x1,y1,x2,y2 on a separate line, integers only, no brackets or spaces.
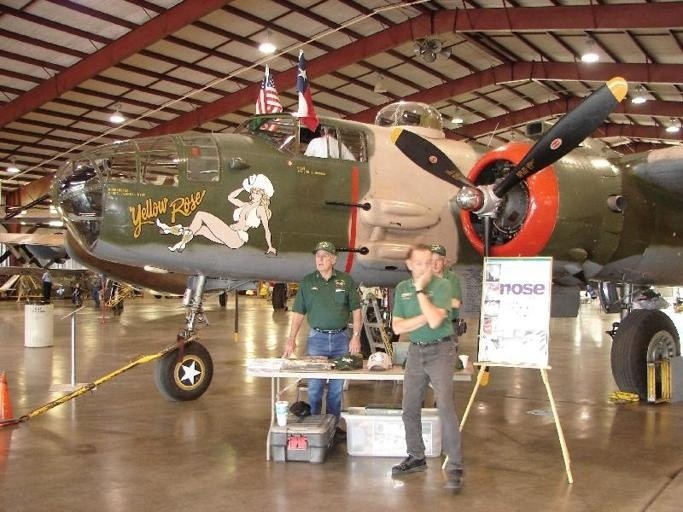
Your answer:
0,426,17,474
0,371,20,428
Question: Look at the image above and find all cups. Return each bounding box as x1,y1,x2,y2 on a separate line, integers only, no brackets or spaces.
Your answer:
275,401,290,427
458,354,469,369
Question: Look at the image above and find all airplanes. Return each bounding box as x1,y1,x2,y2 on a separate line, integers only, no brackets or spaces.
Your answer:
0,231,291,311
46,75,682,409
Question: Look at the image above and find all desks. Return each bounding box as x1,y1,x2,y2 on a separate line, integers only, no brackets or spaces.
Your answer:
246,356,476,462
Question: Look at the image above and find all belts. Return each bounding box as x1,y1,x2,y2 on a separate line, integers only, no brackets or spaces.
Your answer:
314,328,346,335
410,336,450,346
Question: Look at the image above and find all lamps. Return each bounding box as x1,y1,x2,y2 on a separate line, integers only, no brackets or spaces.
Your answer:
6,155,21,173
109,104,125,124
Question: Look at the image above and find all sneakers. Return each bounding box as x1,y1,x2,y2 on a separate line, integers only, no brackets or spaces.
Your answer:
392,455,426,474
335,427,347,440
445,470,462,489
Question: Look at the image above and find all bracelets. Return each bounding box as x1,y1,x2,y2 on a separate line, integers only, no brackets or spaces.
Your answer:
415,289,424,295
352,331,361,336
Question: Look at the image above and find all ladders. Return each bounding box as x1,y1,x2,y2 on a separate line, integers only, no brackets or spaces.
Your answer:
345,292,395,359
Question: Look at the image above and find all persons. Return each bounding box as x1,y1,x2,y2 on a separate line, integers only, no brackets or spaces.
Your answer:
429,243,463,348
40,268,51,304
302,126,358,163
14,274,37,303
153,172,278,256
390,243,465,495
70,283,80,306
281,241,362,442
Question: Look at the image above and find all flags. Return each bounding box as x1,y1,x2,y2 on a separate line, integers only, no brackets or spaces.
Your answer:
294,51,321,133
253,65,283,132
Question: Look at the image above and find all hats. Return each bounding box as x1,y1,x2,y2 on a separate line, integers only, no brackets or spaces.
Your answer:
429,244,446,256
243,174,274,198
367,352,392,371
312,241,336,255
336,354,362,370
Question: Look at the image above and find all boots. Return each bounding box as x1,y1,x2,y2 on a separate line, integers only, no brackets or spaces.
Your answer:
168,229,193,253
157,218,182,236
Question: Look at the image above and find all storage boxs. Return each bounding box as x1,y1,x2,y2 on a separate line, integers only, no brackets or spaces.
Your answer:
339,405,442,458
